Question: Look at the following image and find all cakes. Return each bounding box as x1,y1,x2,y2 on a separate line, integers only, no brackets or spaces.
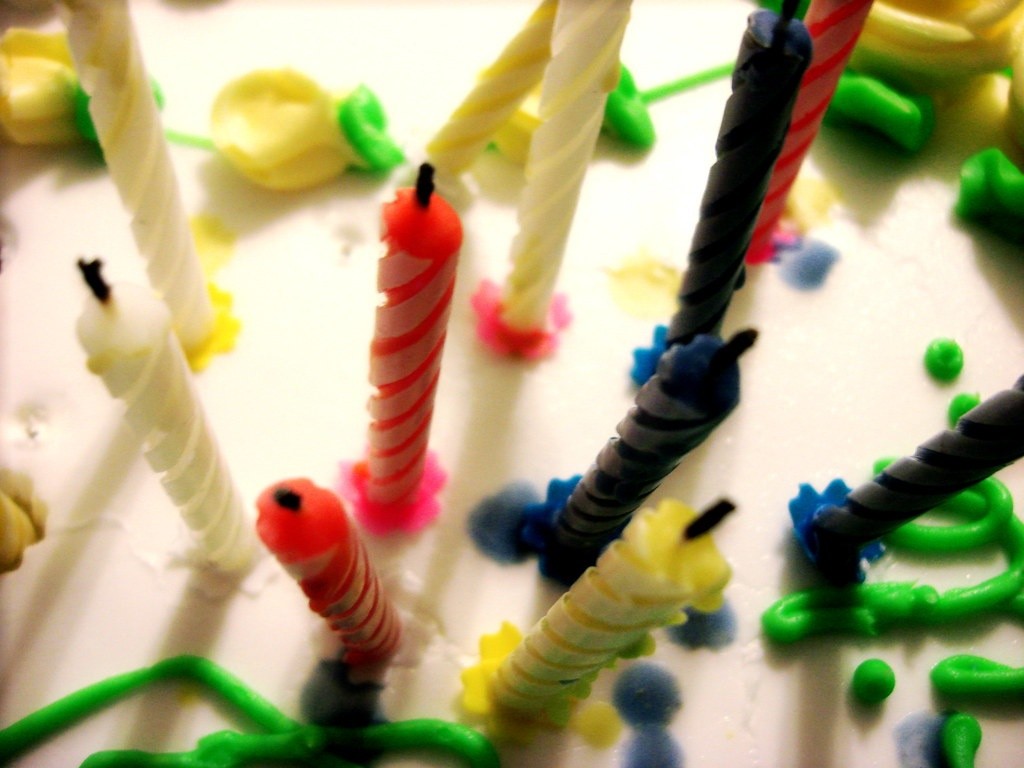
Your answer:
0,0,1024,768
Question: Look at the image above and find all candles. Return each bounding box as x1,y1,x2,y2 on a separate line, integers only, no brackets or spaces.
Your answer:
72,258,256,572
366,163,464,507
490,497,740,723
57,0,218,341
746,0,874,262
497,1,634,330
807,374,1024,568
257,479,404,659
426,0,559,175
664,0,814,347
555,327,761,569
